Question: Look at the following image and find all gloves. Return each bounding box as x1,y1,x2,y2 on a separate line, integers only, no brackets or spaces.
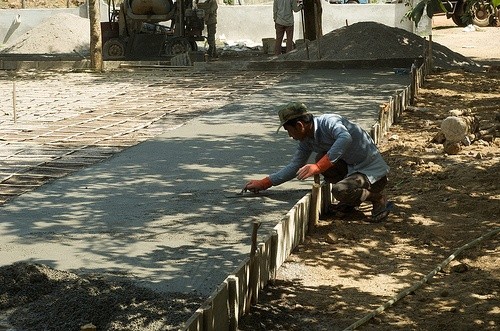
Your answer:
243,175,275,192
296,154,334,180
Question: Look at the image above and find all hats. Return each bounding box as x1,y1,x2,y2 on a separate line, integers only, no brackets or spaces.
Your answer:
275,101,318,133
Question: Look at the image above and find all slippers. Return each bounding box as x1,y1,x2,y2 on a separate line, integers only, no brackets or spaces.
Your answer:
370,200,395,224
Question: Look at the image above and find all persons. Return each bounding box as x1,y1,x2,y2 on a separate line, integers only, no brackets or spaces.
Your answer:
245,101,394,222
273,0,304,55
199,0,219,58
